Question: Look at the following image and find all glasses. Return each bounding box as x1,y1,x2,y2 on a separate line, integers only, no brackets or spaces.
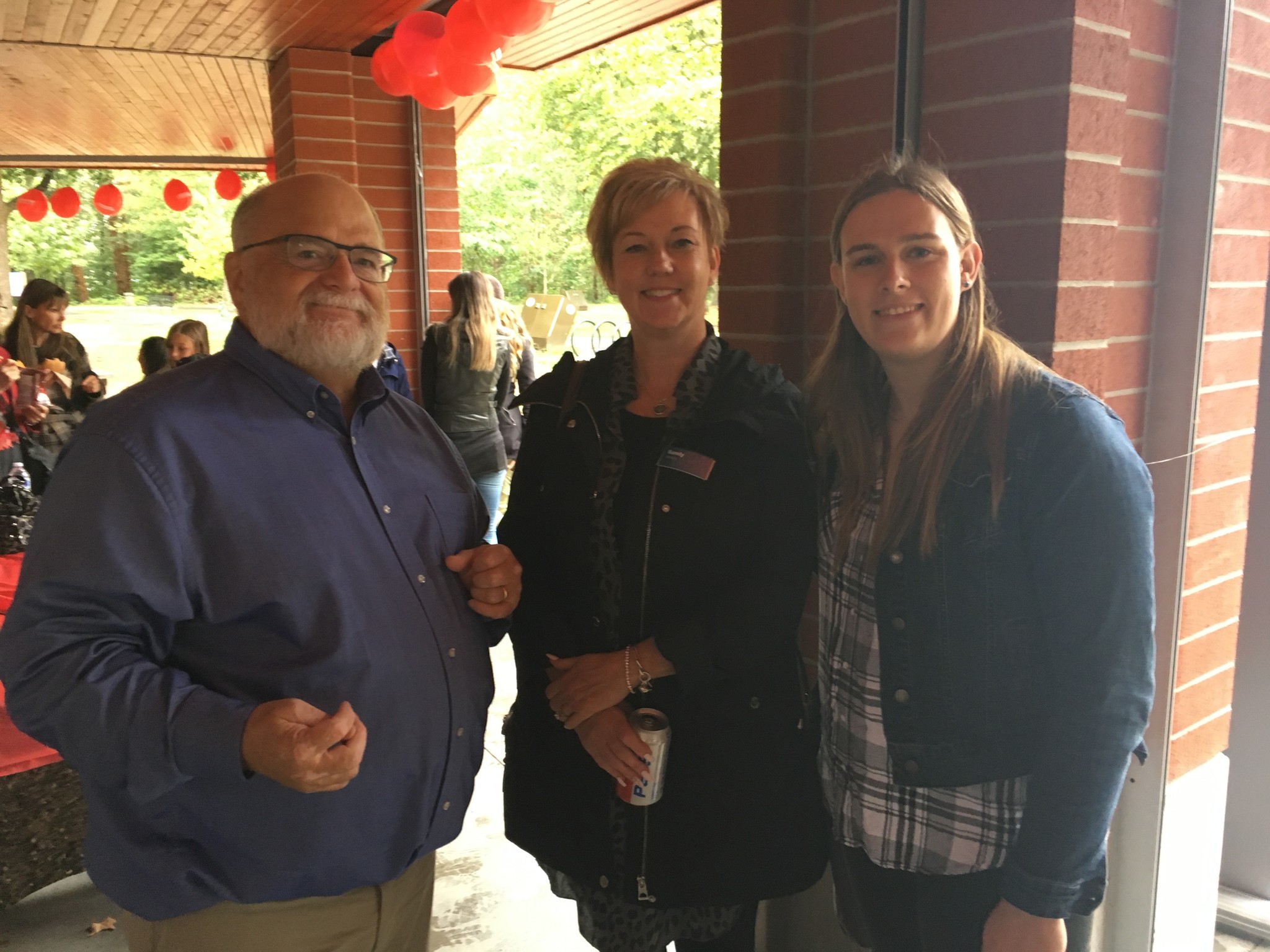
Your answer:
242,232,396,283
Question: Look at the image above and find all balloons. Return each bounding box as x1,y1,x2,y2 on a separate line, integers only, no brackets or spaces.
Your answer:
266,160,277,183
371,0,553,110
164,179,192,212
215,168,241,200
15,188,80,222
93,183,123,215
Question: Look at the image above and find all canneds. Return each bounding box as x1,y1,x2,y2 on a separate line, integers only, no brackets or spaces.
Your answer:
616,708,672,806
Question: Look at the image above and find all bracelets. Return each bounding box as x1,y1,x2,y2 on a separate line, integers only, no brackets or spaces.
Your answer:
633,648,653,693
625,645,637,695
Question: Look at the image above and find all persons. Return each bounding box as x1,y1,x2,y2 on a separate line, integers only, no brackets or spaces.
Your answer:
0,344,49,488
497,158,829,952
0,173,525,951
139,336,171,379
420,270,510,544
6,278,105,470
372,342,411,400
484,272,536,464
805,160,1153,952
167,319,209,370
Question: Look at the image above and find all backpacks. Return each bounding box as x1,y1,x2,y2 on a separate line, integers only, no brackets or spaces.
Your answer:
0,476,40,554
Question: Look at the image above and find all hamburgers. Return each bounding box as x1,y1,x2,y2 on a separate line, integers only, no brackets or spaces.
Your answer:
42,357,66,376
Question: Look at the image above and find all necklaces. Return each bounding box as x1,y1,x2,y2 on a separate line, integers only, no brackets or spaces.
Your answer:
638,383,671,414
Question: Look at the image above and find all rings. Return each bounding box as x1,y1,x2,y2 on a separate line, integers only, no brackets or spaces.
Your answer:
503,588,507,599
554,712,568,721
